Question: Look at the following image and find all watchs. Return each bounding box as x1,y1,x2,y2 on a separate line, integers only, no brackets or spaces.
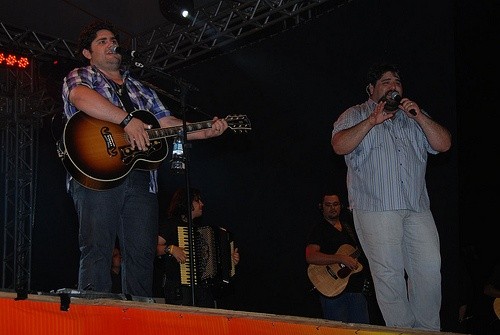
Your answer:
165,245,170,254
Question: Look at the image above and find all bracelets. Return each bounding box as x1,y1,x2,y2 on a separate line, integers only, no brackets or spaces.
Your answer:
120,113,134,128
170,245,173,254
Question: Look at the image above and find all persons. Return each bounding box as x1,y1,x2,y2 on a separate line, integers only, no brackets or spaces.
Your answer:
62,21,227,295
156,187,240,307
305,191,369,324
458,265,500,335
332,64,450,332
111,247,121,293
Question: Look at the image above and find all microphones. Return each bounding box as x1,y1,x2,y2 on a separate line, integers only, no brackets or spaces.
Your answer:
392,92,417,116
112,46,141,58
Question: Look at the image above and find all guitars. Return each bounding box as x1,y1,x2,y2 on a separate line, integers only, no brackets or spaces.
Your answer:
55,108,252,191
307,244,364,298
491,297,500,320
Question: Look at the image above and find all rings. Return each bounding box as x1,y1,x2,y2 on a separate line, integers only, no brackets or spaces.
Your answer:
410,103,413,106
